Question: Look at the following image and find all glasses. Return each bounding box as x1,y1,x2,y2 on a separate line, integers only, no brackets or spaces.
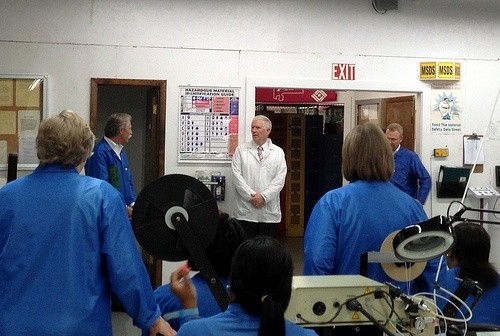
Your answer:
387,136,401,140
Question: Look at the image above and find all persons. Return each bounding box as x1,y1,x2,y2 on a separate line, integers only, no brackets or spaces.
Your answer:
169,236,319,336
83,112,137,217
230,115,287,239
0,110,178,336
301,121,447,315
142,213,254,336
419,221,500,326
384,122,431,206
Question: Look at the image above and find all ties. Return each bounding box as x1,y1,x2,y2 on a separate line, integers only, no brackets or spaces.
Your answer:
258,147,264,160
